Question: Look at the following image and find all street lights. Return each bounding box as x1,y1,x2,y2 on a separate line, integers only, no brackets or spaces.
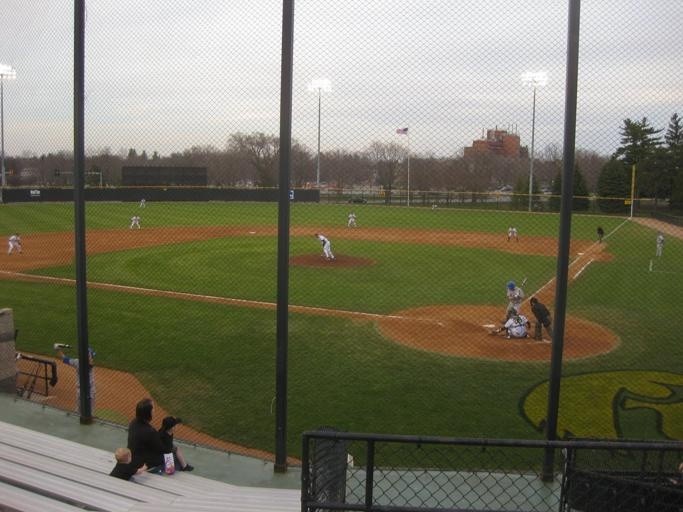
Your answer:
519,68,552,215
308,77,333,188
0,60,18,186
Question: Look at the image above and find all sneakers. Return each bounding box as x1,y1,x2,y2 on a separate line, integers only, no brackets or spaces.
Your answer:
180,463,194,471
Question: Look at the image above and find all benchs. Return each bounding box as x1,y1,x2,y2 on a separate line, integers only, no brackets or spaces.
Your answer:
0,419,332,512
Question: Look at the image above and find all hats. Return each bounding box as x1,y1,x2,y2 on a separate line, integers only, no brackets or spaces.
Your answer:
162,417,181,429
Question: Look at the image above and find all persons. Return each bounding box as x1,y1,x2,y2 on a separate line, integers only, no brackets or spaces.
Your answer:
55,343,95,418
656,232,664,257
495,309,531,339
530,297,553,342
597,226,604,243
314,233,335,262
7,233,23,257
347,211,357,228
140,198,146,209
130,214,141,230
503,280,524,336
109,399,194,481
507,225,519,242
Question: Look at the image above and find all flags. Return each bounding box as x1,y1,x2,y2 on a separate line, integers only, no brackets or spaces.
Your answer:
395,127,409,135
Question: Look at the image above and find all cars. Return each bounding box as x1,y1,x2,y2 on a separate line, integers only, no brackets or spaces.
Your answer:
348,197,367,203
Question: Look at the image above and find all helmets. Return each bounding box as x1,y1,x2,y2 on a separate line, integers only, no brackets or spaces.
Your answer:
507,281,515,289
